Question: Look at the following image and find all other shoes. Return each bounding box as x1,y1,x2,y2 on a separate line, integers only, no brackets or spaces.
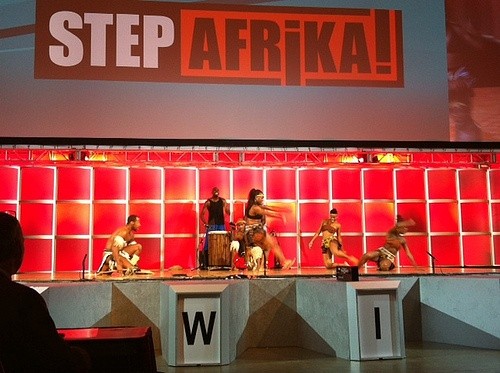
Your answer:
344,261,349,266
283,259,295,270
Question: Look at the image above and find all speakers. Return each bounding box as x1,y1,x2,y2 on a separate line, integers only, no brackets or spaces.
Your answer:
57,326,157,373
337,266,359,281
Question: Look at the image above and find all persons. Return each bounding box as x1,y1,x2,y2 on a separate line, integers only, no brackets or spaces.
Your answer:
199,186,231,270
243,188,296,269
356,214,425,271
233,219,247,241
308,207,364,268
0,210,95,373
97,214,143,277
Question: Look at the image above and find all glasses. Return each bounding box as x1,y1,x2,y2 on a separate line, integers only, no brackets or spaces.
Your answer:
257,195,265,199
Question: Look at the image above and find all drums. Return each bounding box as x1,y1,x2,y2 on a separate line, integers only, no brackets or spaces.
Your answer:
207,231,232,269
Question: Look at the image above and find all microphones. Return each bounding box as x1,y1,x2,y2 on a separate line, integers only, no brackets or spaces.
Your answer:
81,255,88,281
427,251,439,261
263,251,266,276
